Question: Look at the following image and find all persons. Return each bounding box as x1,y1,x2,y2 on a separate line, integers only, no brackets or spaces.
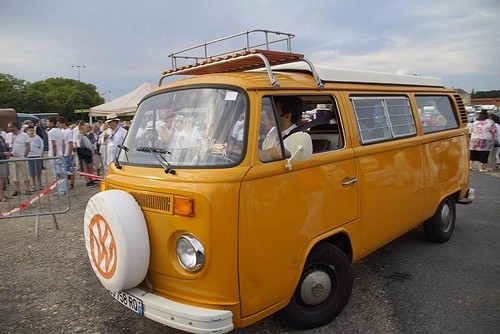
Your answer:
0,131,14,202
231,95,313,162
69,117,133,188
21,119,45,194
45,116,70,198
0,120,32,197
490,115,500,169
468,109,498,174
56,116,75,189
418,107,447,127
139,111,209,150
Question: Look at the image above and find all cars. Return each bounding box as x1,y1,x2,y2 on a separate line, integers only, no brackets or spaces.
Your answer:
466,104,500,124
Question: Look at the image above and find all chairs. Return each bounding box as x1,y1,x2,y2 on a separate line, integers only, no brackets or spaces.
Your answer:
307,130,340,153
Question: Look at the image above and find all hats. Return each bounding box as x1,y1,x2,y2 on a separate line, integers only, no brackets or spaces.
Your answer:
105,113,120,123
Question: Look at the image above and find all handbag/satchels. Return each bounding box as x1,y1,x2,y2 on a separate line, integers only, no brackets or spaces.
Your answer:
78,146,93,158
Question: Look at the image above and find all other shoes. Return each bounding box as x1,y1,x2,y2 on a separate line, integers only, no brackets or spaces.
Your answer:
86,181,100,187
30,186,37,193
69,184,75,189
25,190,31,195
5,196,13,199
12,190,22,196
1,198,8,202
40,185,47,190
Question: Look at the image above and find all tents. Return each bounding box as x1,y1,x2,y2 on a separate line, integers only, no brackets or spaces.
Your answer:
88,81,157,126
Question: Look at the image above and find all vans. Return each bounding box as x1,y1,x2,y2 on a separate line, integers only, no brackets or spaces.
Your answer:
82,27,477,334
16,113,59,132
